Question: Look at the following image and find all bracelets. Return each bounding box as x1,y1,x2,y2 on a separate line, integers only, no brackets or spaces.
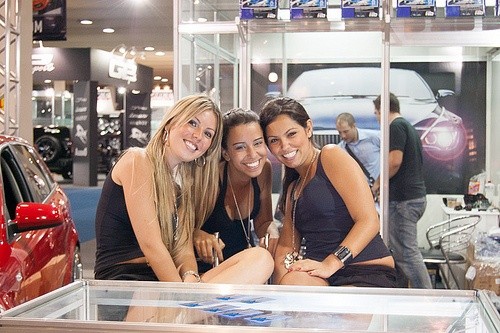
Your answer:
331,244,354,269
182,271,201,282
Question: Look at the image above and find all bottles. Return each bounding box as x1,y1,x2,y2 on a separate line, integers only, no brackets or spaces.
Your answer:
485,181,494,202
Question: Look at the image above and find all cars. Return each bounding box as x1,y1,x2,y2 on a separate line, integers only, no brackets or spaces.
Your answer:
264,67,471,196
0,134,83,313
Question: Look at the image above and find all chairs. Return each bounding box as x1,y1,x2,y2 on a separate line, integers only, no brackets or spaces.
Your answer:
416,214,481,290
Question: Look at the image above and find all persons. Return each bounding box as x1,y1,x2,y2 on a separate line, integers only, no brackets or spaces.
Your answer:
370,92,433,289
259,98,398,287
193,108,279,274
335,113,380,212
93,95,276,321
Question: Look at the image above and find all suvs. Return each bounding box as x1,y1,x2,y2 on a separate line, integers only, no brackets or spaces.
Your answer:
33,126,74,178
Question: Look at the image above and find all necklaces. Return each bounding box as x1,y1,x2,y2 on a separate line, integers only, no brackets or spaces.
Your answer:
283,152,317,271
228,173,252,248
168,167,183,240
